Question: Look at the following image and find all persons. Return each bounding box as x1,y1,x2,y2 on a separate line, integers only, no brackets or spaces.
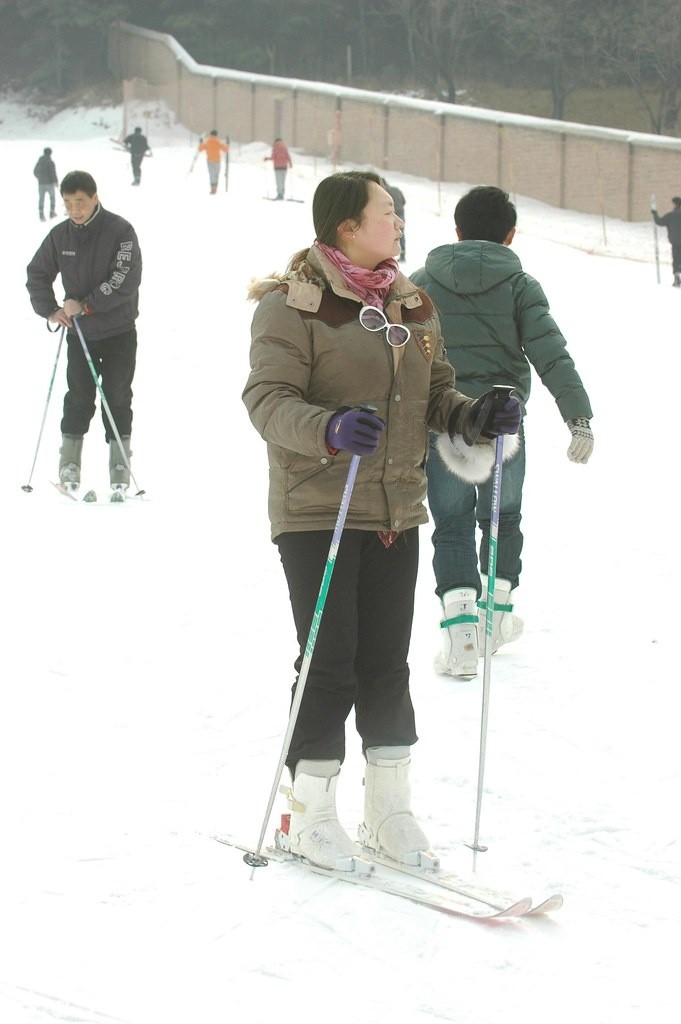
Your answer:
263,137,293,201
408,184,596,684
24,169,143,494
32,147,58,222
380,177,407,263
651,196,681,288
123,126,151,186
239,169,523,874
197,129,231,195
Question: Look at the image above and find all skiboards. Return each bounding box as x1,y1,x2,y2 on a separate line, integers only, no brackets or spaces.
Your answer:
109,137,153,158
190,132,230,192
49,475,124,502
263,196,304,203
210,811,568,918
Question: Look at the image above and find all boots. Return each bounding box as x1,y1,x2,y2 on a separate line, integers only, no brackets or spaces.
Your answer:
366,754,430,866
478,574,524,657
109,435,132,491
434,587,479,679
58,434,84,489
290,768,365,871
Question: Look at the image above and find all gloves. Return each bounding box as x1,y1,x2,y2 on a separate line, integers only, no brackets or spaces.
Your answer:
328,407,385,455
567,417,594,464
471,396,521,439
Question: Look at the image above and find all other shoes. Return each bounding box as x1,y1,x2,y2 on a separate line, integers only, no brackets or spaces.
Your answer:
131,179,139,185
49,212,57,218
209,186,217,194
672,275,681,287
40,215,46,222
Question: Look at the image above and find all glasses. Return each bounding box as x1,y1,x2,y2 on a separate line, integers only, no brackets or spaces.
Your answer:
359,306,411,348
